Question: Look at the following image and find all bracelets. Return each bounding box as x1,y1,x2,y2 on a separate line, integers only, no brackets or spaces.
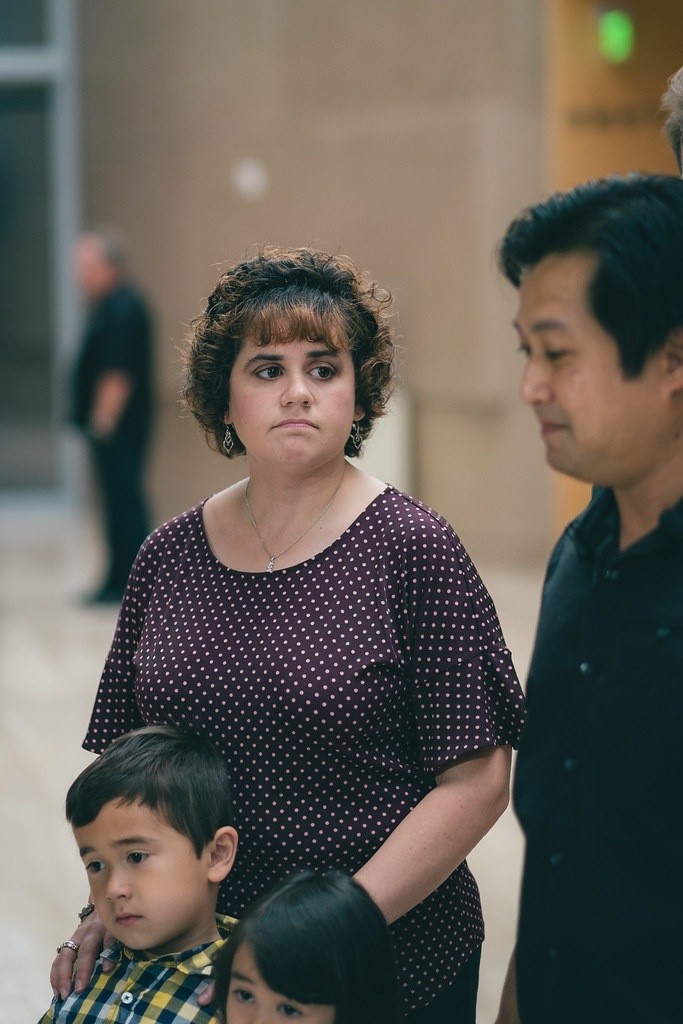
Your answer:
78,900,96,921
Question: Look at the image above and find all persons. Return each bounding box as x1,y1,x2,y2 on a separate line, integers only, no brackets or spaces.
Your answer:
37,727,243,1024
498,69,683,1023
51,240,527,1024
60,223,160,605
210,867,390,1024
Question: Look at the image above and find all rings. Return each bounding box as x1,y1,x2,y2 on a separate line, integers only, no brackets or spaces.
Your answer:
56,941,80,953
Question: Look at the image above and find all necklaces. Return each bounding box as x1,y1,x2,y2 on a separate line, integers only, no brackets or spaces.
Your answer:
236,464,355,574
